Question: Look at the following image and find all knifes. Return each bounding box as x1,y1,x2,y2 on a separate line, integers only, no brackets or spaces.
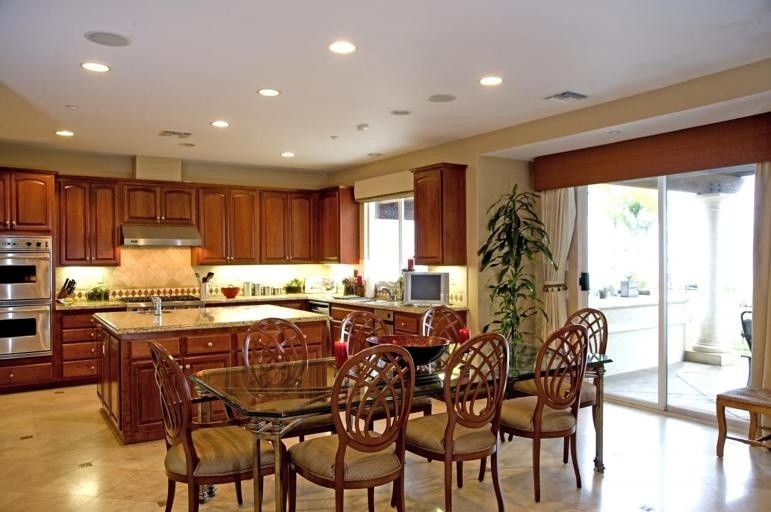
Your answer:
63,278,76,295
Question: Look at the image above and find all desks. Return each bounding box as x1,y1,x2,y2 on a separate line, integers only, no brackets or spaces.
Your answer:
185,340,614,512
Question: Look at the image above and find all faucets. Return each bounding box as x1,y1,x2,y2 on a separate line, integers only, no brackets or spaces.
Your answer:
376,287,397,301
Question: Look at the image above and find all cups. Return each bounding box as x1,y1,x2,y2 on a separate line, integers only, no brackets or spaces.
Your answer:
354,285,364,297
200,282,209,300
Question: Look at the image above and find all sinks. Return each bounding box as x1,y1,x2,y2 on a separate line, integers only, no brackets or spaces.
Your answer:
356,299,404,308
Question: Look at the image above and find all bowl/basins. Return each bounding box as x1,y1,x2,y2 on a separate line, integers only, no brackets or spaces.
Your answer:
221,287,241,299
364,334,451,367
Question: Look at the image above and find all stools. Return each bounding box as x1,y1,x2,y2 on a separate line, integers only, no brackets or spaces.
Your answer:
716,385,771,458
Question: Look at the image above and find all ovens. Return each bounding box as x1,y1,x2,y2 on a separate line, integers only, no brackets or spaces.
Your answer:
0,236,54,360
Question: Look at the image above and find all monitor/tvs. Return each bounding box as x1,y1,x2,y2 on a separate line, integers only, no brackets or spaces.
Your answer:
403,272,449,307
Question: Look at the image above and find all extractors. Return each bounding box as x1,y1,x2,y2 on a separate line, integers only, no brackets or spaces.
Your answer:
121,157,202,248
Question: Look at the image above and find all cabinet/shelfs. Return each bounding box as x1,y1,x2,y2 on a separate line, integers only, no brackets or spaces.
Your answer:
408,162,469,266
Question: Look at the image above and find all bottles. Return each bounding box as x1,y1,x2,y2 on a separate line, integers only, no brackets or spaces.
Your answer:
244,282,281,296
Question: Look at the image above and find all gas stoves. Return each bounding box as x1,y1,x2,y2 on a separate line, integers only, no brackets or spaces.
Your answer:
120,294,205,311
